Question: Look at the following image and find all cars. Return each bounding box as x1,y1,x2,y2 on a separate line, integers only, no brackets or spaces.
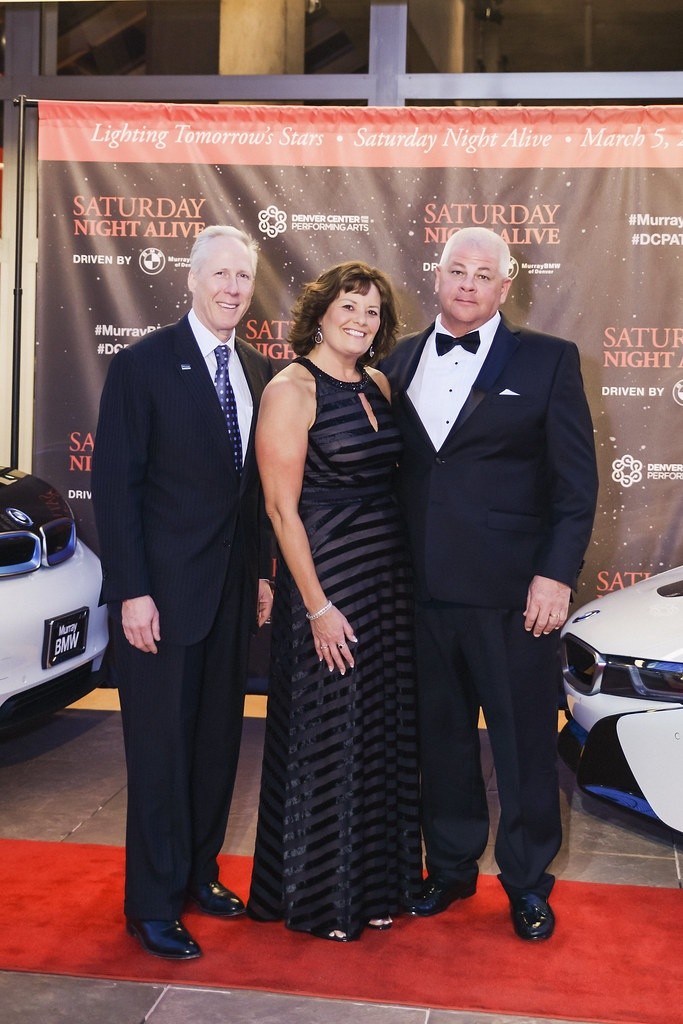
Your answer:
555,562,683,849
1,463,111,747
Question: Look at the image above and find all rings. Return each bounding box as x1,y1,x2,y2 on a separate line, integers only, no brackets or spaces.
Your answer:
320,646,327,650
550,614,559,618
338,643,346,649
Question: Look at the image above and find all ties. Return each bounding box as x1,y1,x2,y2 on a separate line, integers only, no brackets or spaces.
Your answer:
213,345,242,487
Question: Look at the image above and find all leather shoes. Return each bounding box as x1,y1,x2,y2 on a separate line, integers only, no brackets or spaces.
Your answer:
406,877,476,916
186,881,245,915
511,893,555,941
125,917,201,960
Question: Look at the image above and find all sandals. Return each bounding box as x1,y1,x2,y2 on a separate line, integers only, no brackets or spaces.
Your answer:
312,924,352,942
365,912,395,929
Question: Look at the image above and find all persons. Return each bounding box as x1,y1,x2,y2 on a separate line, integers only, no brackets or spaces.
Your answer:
92,226,273,961
375,228,598,940
257,261,423,942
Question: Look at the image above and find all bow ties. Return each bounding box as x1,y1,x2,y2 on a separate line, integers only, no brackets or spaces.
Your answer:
435,331,481,357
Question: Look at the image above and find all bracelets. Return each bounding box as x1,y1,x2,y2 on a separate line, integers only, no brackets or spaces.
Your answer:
306,599,332,620
